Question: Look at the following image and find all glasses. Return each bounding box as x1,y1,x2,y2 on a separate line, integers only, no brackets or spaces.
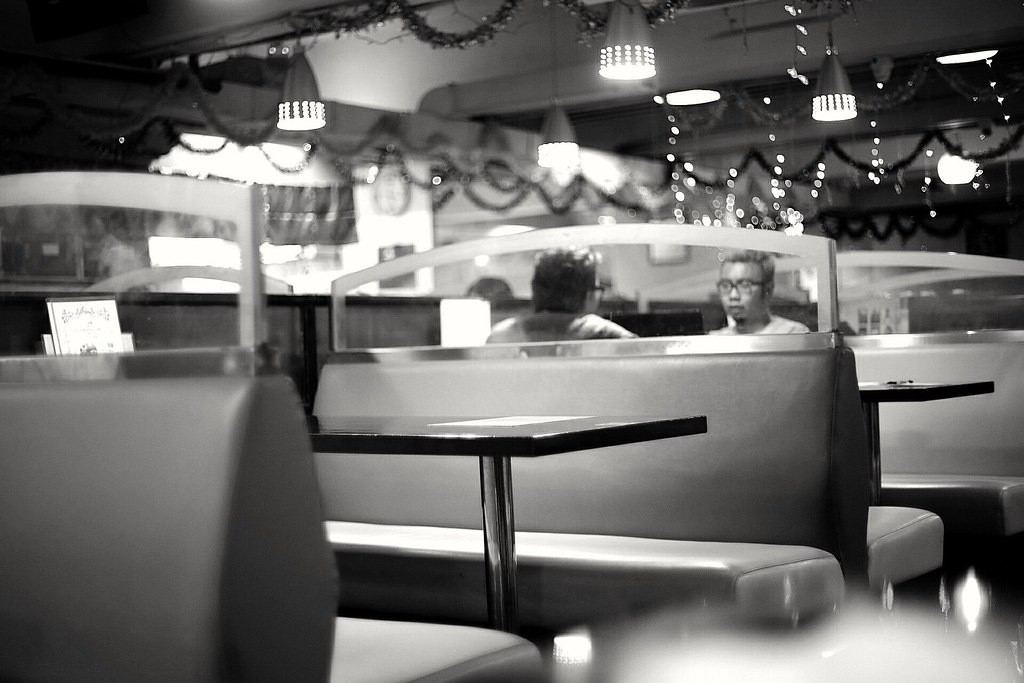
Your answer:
595,286,605,297
717,279,762,294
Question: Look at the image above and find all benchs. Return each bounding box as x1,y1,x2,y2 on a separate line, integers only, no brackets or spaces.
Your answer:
0,172,546,683
639,250,1024,538
308,224,945,633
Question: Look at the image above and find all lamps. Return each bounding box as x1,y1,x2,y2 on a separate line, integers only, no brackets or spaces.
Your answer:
811,15,858,122
276,35,326,131
538,0,579,168
597,0,657,80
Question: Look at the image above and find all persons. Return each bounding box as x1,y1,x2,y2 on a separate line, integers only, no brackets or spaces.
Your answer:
710,250,811,335
486,247,634,340
467,275,511,299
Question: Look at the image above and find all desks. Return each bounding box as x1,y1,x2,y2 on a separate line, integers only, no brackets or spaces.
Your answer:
310,417,707,633
858,382,994,505
